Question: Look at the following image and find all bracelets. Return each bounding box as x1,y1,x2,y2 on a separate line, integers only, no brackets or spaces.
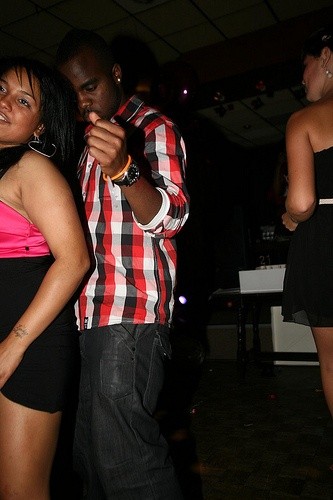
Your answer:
103,155,131,181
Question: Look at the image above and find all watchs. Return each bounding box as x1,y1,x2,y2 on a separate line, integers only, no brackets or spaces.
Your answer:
111,159,140,187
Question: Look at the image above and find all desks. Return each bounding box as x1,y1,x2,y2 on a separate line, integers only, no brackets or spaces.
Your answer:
212,287,284,382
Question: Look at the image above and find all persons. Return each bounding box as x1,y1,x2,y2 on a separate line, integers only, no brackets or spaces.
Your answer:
282,33,333,417
0,56,91,500
53,28,190,500
